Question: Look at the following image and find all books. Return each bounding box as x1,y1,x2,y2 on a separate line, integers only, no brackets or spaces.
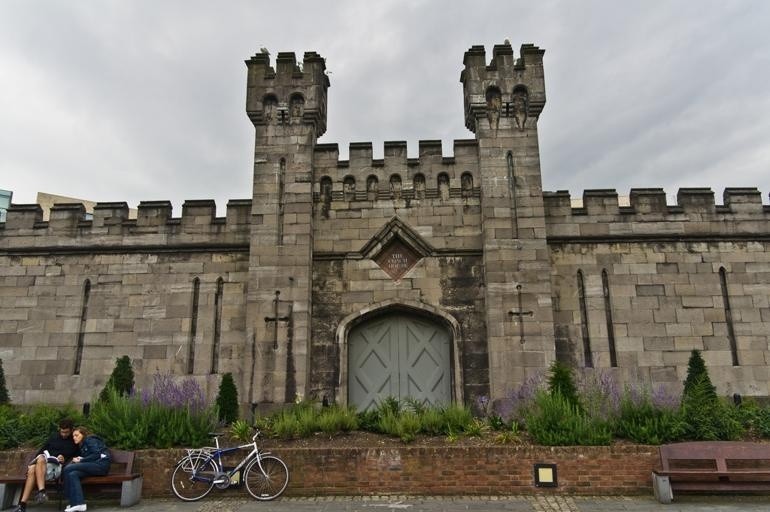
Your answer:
43,450,60,465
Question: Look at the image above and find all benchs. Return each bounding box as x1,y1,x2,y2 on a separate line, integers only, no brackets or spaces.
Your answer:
0,451,143,511
651,441,770,504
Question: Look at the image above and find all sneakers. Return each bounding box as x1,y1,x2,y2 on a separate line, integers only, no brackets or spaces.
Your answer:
15,502,26,512
35,489,46,504
64,504,87,512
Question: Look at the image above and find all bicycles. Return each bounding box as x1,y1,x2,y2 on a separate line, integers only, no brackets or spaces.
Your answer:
171,425,290,503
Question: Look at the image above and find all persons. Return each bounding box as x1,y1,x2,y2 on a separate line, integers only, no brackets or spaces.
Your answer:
62,426,112,511
13,416,80,512
